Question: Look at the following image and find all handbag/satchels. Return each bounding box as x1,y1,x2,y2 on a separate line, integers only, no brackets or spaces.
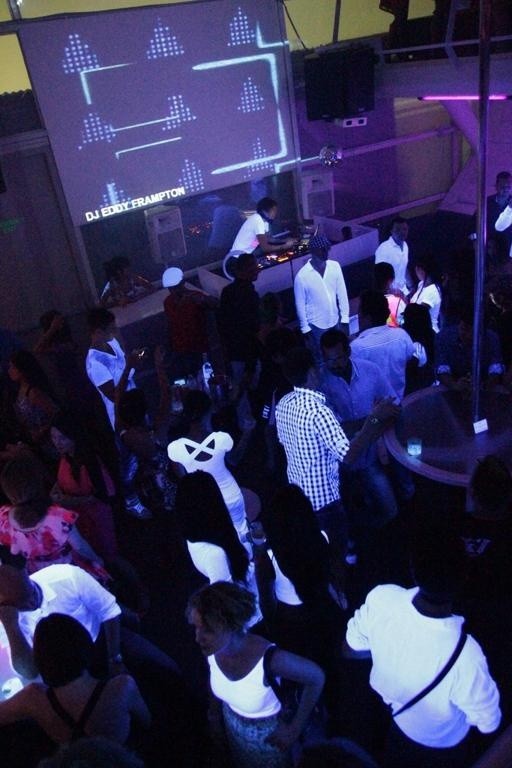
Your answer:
281,682,329,744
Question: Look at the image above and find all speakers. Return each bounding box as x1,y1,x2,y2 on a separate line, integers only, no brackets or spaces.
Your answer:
303,47,375,122
144,206,187,265
297,168,335,220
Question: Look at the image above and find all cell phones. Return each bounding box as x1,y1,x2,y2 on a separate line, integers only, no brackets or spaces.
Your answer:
473,418,489,434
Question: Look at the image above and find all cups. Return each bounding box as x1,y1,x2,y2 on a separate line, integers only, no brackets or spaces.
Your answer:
249,521,266,546
405,438,423,456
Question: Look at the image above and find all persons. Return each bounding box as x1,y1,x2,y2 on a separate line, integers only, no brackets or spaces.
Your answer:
208,188,241,250
229,197,277,256
477,171,510,224
2,220,510,767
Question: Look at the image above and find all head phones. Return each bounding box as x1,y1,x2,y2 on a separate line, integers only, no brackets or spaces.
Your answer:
257,209,273,224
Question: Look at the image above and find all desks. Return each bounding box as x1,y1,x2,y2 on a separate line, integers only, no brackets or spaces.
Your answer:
380,385,510,512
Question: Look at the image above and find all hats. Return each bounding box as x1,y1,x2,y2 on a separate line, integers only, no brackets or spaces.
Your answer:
161,267,184,288
309,234,331,248
